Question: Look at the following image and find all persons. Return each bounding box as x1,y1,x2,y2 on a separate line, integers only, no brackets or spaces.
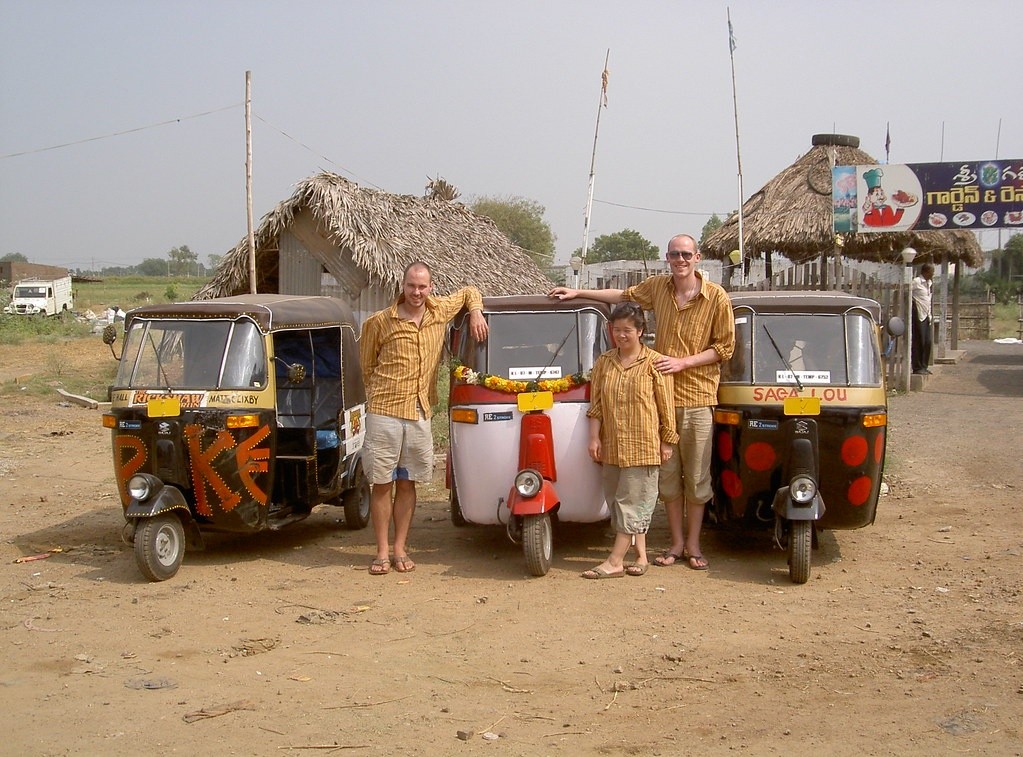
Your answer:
548,234,734,570
904,264,935,375
582,302,681,579
361,262,489,575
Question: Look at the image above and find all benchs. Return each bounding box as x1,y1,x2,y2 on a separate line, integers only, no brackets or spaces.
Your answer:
274,377,361,449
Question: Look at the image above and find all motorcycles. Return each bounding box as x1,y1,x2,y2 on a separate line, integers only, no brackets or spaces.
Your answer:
712,290,890,584
445,294,621,578
103,293,372,583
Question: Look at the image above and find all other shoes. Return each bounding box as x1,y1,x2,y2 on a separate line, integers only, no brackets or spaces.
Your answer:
913,369,928,375
924,369,932,374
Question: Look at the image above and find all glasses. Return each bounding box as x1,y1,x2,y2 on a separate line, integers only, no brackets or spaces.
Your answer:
669,251,697,261
616,302,641,310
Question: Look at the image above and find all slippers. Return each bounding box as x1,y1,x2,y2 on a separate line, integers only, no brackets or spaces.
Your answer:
368,559,391,574
628,562,649,575
687,555,709,569
653,552,685,565
393,556,415,572
584,567,624,578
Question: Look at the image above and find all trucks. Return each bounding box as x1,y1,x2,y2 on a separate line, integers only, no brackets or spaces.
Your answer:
10,276,77,318
